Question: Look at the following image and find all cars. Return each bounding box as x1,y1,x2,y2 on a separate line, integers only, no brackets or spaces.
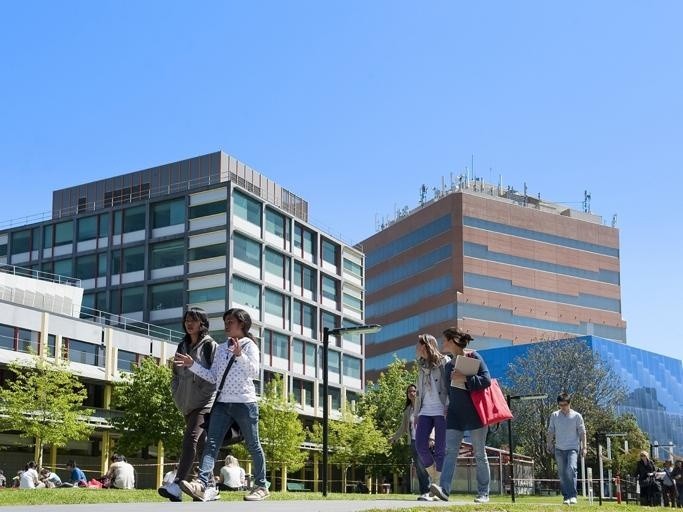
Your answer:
345,480,365,493
267,476,302,490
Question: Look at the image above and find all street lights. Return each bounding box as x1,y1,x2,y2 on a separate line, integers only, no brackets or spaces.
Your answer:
596,430,628,505
650,443,677,457
321,322,383,497
506,391,549,503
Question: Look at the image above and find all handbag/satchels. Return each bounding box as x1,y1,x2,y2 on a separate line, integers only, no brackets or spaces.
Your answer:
199,412,244,446
470,379,513,426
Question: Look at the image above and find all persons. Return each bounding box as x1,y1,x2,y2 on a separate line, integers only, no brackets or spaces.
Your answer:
388,384,430,501
429,327,492,503
633,450,682,509
157,306,221,502
171,308,270,501
0,453,248,491
544,393,588,505
411,333,451,502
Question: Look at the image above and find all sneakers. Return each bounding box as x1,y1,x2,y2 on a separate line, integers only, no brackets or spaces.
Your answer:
418,483,576,504
159,478,271,502
